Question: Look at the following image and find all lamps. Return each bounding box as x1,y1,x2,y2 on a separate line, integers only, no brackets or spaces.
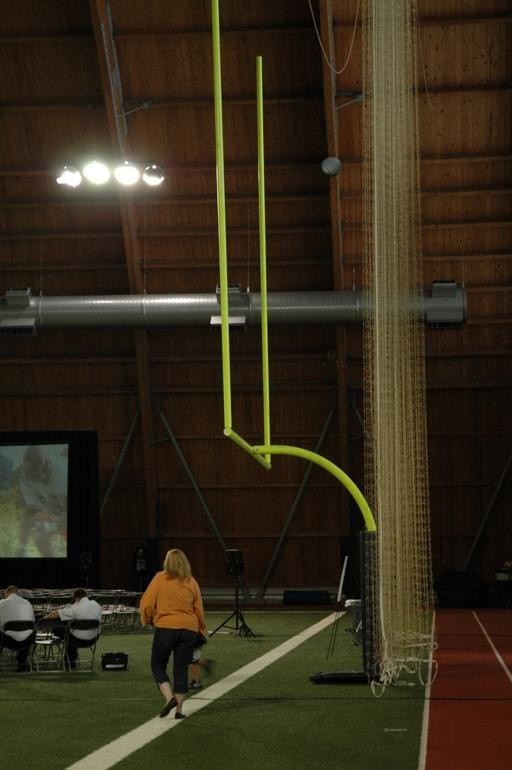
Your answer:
321,157,341,176
56,159,164,187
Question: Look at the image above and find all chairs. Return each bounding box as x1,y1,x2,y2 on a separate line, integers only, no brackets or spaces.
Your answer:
344,618,363,645
0,588,143,675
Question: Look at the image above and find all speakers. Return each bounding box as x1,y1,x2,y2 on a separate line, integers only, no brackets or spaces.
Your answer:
78,552,92,571
225,549,245,575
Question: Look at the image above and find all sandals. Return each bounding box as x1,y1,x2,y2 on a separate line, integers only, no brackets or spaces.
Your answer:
159,698,185,719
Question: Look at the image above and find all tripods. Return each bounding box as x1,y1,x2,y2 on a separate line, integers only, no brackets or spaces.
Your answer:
208,575,256,638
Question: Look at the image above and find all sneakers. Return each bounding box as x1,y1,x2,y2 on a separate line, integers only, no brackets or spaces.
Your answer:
187,680,203,689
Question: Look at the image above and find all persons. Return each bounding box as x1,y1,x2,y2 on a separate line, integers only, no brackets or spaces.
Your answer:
0,584,35,670
190,639,215,689
36,589,102,669
140,548,208,720
